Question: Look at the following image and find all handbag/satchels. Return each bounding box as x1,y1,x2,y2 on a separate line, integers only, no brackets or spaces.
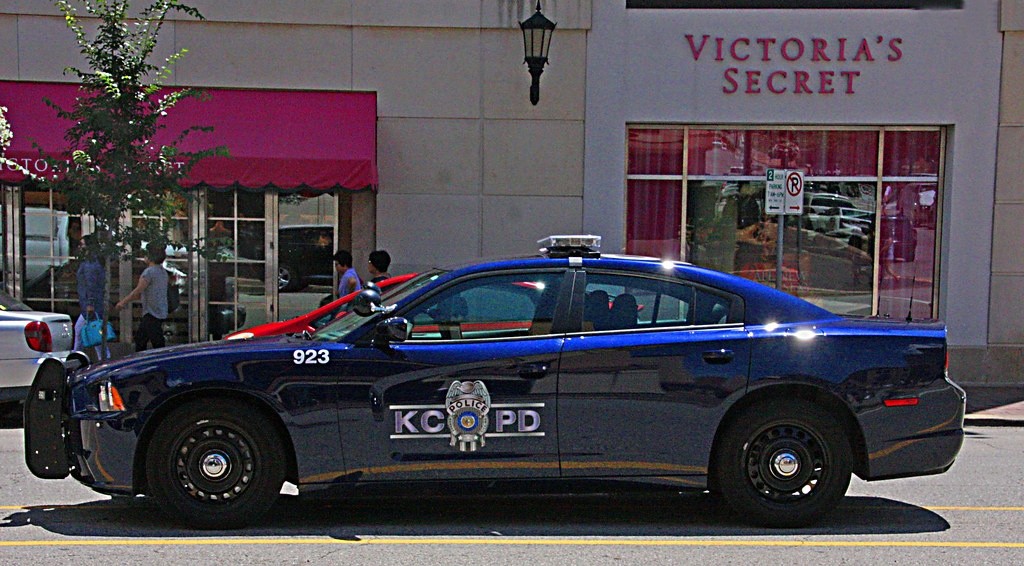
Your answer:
80,310,117,348
168,274,180,313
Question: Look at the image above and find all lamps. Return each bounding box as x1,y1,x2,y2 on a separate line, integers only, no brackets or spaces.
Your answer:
517,0,559,105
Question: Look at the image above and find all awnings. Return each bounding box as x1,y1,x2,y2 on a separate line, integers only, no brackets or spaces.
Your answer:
0,81,378,190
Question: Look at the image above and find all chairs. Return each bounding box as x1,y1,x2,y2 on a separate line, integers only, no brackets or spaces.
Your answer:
601,293,639,333
583,288,612,326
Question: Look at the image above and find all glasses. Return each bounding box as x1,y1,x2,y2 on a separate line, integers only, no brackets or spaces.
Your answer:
369,260,371,263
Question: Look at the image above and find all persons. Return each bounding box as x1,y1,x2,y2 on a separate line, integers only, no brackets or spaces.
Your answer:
115,244,170,352
367,250,391,283
74,235,111,361
879,219,900,282
334,249,361,298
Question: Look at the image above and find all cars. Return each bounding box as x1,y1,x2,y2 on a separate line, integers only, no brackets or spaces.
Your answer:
715,166,939,295
0,202,340,354
23,233,969,534
215,265,646,345
0,287,74,417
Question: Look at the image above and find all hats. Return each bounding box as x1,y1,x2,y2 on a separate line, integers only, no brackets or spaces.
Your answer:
370,250,390,272
331,249,352,268
